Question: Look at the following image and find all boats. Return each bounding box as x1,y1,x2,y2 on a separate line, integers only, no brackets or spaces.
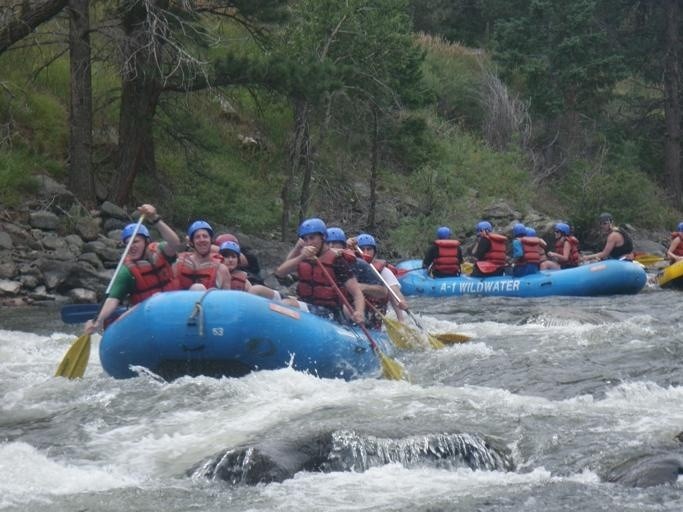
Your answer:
394,255,648,298
649,260,683,289
96,288,399,386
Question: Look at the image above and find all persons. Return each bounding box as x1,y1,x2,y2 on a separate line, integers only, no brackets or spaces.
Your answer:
667,222,683,262
422,213,634,277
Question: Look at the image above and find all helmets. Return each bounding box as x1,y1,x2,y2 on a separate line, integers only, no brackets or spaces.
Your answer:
512,223,536,237
297,218,329,241
677,222,683,231
218,240,240,257
436,226,450,239
121,223,150,244
475,221,493,232
212,233,239,246
324,227,347,245
554,223,570,235
356,233,377,253
599,212,614,224
187,220,215,242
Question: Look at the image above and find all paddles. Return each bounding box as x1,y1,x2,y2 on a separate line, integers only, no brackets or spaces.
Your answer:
59,303,131,323
313,255,412,384
635,254,673,265
356,245,445,351
366,299,424,351
462,260,513,276
55,212,146,379
397,267,426,275
429,332,472,345
